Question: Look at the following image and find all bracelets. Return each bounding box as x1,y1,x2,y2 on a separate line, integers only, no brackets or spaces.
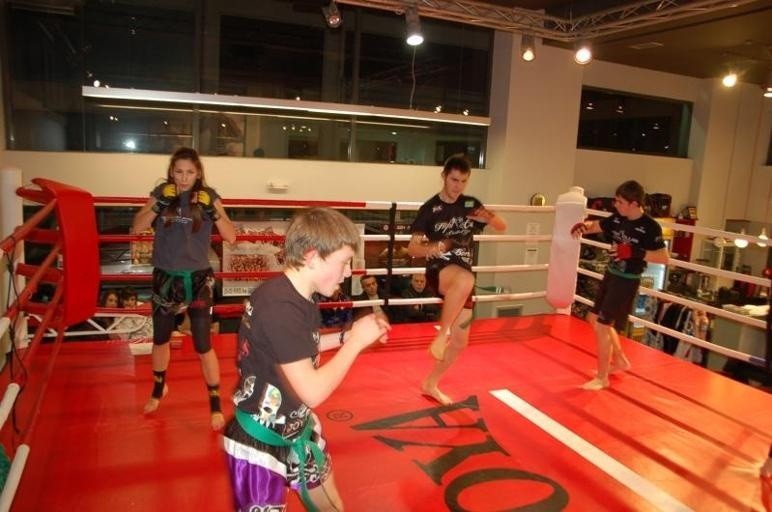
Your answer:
338,330,346,346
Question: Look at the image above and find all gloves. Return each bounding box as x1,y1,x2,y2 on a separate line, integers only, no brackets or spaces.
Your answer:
149,182,181,214
196,189,222,224
615,240,646,263
570,223,588,239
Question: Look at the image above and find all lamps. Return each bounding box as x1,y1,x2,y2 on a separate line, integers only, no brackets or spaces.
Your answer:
711,84,767,250
319,2,342,28
81,84,491,126
721,71,771,97
518,26,539,63
570,38,597,67
401,6,425,47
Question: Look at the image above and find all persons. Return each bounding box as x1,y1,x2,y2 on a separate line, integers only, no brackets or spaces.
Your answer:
87,287,120,342
130,146,238,432
569,177,674,392
221,204,394,511
120,286,138,309
352,275,396,327
405,156,508,408
395,272,440,324
316,284,355,330
